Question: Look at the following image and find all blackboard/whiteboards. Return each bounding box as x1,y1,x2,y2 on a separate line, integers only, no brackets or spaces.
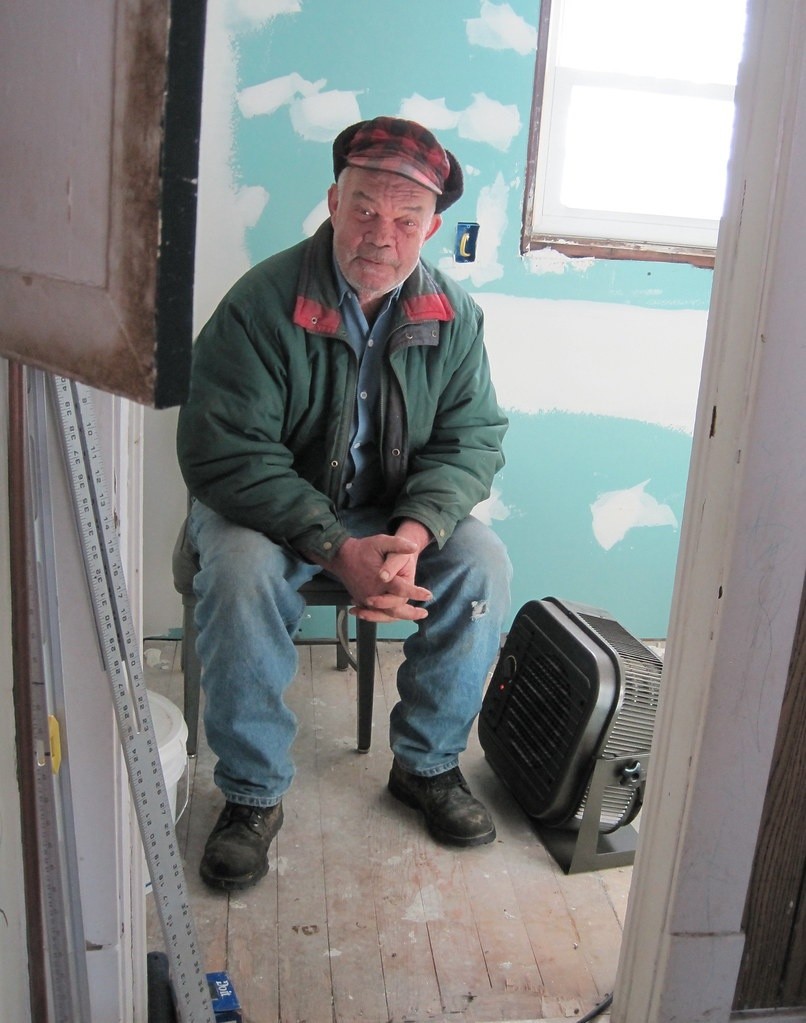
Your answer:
519,1,754,270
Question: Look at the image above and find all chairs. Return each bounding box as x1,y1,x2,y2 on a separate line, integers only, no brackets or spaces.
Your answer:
171,484,377,755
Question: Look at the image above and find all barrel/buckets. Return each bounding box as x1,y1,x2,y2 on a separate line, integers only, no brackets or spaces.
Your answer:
141,687,190,892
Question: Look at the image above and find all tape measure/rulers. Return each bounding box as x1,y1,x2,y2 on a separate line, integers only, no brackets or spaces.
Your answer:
47,370,218,1023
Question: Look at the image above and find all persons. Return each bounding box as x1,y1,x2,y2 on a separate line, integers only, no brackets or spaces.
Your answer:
171,117,513,890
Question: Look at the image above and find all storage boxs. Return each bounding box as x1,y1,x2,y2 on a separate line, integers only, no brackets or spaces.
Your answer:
206,971,243,1023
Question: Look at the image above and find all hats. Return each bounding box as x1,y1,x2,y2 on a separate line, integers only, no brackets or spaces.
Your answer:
341,116,451,196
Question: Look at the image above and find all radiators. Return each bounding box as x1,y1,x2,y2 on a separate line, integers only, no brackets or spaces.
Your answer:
476,597,662,875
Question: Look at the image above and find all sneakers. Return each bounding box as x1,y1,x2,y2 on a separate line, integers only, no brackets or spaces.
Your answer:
199,800,284,890
388,757,497,846
